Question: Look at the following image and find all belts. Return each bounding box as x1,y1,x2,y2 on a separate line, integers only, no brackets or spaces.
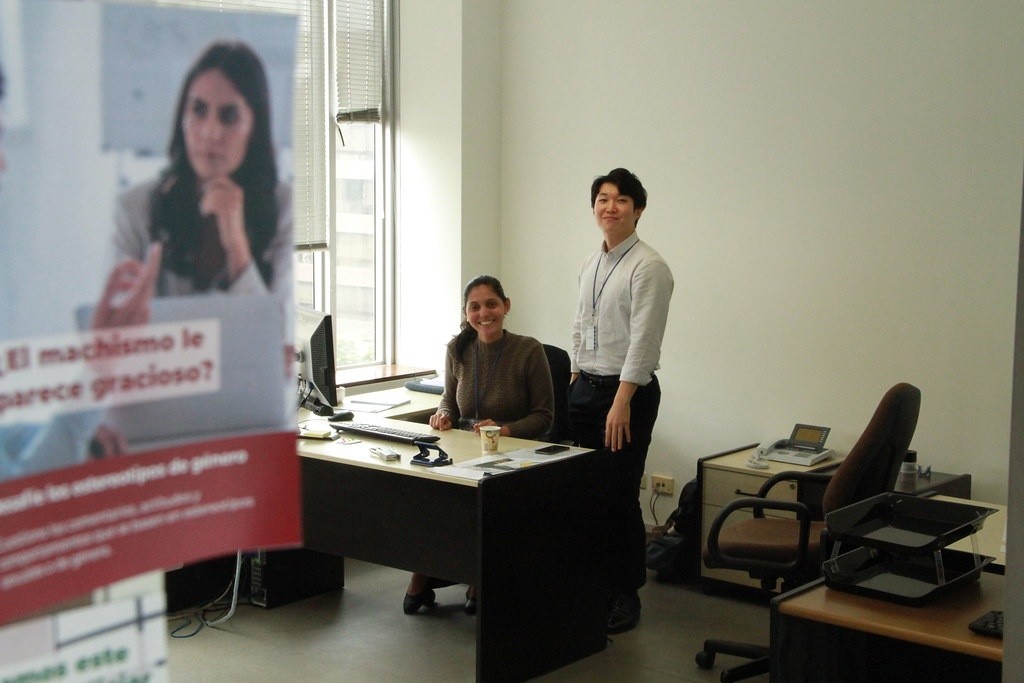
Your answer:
580,370,621,388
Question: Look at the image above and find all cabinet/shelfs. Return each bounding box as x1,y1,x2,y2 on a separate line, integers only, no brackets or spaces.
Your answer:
696,443,849,608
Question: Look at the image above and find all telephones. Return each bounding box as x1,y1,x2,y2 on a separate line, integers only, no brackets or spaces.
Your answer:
756,423,833,466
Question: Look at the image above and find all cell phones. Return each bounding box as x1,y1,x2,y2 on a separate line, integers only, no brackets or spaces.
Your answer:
535,445,569,455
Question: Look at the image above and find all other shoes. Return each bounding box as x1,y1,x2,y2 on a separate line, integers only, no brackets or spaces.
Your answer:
465,588,477,609
403,568,428,615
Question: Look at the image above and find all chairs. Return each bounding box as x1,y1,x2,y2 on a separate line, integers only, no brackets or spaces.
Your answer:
694,383,920,683
543,340,574,413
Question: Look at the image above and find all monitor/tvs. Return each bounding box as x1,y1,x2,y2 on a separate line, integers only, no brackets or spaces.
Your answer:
292,305,338,416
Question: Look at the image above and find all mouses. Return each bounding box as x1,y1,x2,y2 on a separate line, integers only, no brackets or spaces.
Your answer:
328,411,354,422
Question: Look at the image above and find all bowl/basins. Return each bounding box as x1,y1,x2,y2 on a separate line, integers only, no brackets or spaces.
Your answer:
337,387,345,404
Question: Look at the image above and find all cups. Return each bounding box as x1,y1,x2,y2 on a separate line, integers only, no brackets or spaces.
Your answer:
480,426,500,454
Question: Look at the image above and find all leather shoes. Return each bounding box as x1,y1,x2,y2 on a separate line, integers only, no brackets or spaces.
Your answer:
607,587,642,634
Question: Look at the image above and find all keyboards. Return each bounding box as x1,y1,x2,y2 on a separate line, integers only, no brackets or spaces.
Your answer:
330,422,440,446
969,610,1003,639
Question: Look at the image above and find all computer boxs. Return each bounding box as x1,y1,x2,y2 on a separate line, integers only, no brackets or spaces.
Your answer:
249,547,344,610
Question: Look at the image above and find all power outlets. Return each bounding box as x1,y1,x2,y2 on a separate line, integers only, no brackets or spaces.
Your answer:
651,476,673,494
640,474,647,488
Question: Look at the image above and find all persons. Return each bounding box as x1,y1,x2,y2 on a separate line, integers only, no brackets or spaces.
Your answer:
403,277,555,614
559,169,673,630
0,40,291,483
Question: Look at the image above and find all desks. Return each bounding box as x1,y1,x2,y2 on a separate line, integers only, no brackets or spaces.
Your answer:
796,465,973,521
238,386,609,683
770,495,1007,683
